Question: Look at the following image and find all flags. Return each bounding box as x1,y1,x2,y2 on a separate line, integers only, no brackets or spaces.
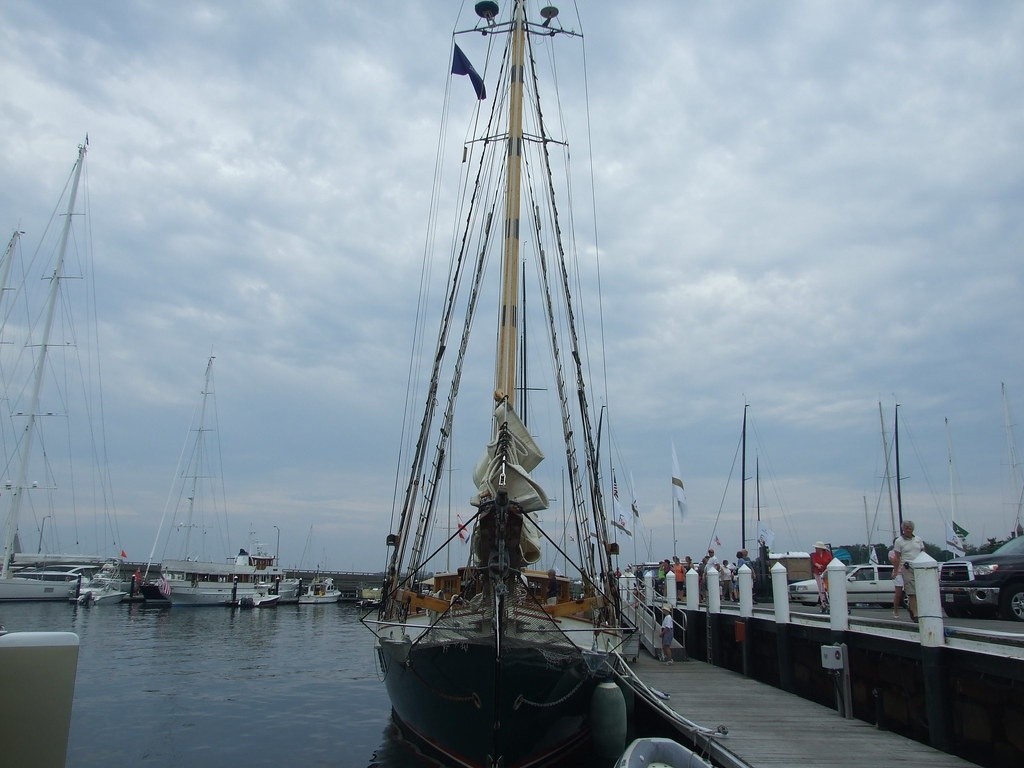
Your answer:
953,522,969,542
613,471,639,533
451,42,487,100
671,442,688,522
457,513,470,544
945,521,965,557
162,574,171,595
869,548,879,565
122,550,127,557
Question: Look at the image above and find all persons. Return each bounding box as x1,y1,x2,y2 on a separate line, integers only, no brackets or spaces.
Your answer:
811,541,832,613
658,605,673,663
721,549,756,602
546,569,557,621
624,567,635,577
698,549,720,603
513,567,528,618
891,520,925,623
659,559,674,595
634,566,643,587
615,567,622,577
643,566,658,590
888,538,904,618
673,556,695,601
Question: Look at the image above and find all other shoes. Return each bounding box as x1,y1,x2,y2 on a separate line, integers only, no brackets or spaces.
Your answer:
892,611,899,619
665,658,674,666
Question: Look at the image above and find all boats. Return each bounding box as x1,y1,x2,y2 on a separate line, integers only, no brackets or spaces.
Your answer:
69,560,127,605
355,598,381,610
298,585,341,605
236,594,280,608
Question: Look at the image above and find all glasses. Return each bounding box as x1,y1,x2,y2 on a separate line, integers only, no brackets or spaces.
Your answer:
710,552,714,554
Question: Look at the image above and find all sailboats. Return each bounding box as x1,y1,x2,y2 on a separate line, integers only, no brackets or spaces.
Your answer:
369,2,635,767
0,135,302,609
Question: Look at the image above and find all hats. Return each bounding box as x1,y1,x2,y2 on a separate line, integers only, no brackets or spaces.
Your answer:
812,541,828,551
661,604,672,612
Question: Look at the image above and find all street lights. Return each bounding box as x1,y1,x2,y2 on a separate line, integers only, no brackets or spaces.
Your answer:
38,515,52,554
273,526,281,565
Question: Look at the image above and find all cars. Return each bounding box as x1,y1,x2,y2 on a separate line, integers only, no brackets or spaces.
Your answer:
939,534,1023,622
787,564,898,608
